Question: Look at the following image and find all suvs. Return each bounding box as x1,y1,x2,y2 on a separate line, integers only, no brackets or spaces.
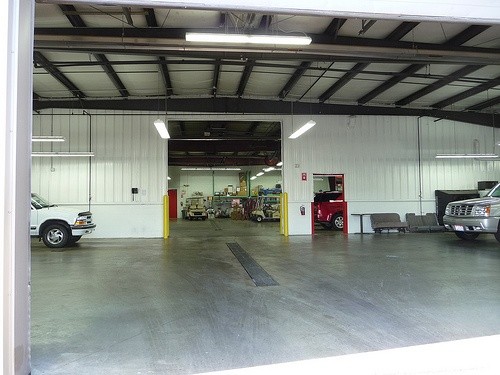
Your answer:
313,188,344,231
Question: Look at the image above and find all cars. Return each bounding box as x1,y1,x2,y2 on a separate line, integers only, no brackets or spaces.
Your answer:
30,189,97,249
441,181,500,244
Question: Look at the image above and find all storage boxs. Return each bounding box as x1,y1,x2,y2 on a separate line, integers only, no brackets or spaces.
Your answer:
215,180,247,196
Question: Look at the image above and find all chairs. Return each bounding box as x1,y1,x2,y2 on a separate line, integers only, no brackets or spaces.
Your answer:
406,213,445,232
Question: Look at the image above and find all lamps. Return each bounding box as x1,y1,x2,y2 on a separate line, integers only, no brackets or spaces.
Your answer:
288,76,316,139
181,161,282,181
154,68,172,140
185,29,311,46
32,91,96,159
435,104,500,162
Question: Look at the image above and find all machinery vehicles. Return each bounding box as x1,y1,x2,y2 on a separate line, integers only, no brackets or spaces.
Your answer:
250,195,281,223
180,192,207,221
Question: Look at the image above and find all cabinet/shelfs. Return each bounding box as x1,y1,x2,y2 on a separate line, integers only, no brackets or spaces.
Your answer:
212,196,247,210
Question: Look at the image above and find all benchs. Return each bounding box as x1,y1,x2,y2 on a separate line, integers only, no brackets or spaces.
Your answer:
370,213,407,236
